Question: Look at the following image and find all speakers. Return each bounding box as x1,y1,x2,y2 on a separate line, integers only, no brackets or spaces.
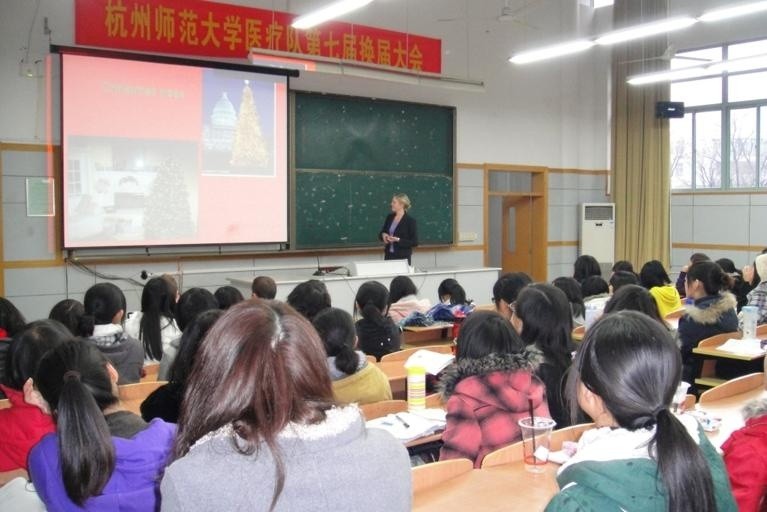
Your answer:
654,101,685,119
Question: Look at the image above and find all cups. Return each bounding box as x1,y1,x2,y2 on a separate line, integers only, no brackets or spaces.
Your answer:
742,306,756,339
518,418,555,473
669,382,691,415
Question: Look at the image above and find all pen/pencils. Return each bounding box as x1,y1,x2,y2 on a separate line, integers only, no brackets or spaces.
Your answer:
394,415,409,430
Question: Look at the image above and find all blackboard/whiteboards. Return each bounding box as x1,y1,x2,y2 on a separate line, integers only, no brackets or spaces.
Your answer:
290,88,458,249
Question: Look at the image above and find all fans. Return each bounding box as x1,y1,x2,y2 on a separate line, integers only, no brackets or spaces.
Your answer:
435,0,546,33
620,42,712,64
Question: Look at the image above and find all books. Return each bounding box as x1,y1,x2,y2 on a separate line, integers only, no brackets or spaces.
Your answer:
716,338,766,358
403,349,456,376
364,408,447,443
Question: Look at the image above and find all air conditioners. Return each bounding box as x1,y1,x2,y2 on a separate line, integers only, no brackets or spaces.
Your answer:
577,203,616,285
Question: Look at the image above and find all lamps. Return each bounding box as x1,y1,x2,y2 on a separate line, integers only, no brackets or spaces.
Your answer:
247,47,486,95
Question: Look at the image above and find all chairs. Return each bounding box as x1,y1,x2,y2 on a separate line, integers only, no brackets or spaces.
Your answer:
699,333,743,377
142,363,161,375
482,422,595,468
366,354,374,360
1,398,11,409
380,345,454,363
412,458,474,494
665,307,685,321
700,373,765,404
427,391,441,409
361,399,408,422
682,394,696,408
476,304,498,312
119,381,168,400
757,323,766,337
401,330,441,344
572,325,586,334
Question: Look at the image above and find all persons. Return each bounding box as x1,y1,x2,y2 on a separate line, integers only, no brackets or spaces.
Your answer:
353,280,403,362
139,309,224,425
437,278,475,308
378,192,418,266
157,287,219,379
544,310,737,512
611,260,633,274
251,276,277,300
214,286,244,310
572,255,601,285
79,282,144,385
678,261,738,394
640,260,681,317
123,274,183,366
387,275,431,322
676,248,767,327
602,284,670,331
0,319,74,476
508,283,589,430
50,299,84,339
719,391,767,511
287,279,331,322
312,307,393,406
0,296,25,399
581,276,608,301
551,276,602,329
159,298,413,512
26,339,178,512
491,271,532,320
589,270,638,324
439,310,550,466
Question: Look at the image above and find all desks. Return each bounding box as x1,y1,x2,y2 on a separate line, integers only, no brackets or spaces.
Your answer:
366,406,447,448
693,333,766,361
410,384,766,512
116,360,435,417
224,267,502,321
